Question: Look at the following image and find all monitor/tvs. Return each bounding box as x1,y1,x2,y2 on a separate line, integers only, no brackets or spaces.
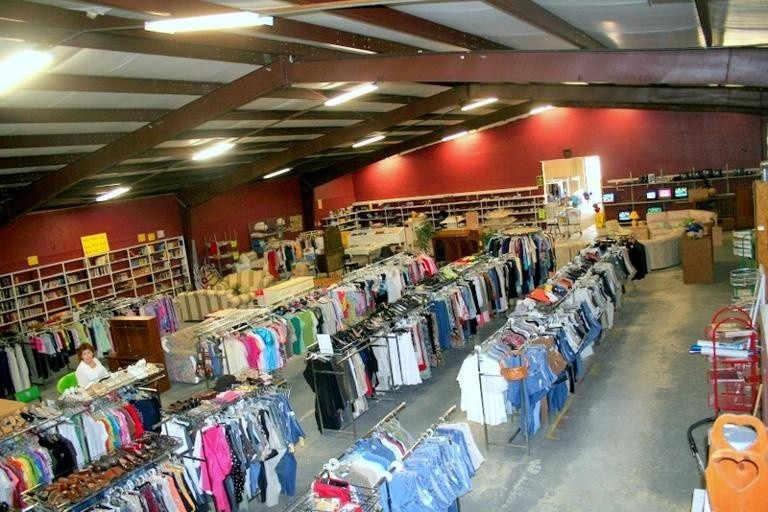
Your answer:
658,188,672,199
646,191,657,200
602,193,616,204
617,210,633,222
674,186,688,199
646,207,663,213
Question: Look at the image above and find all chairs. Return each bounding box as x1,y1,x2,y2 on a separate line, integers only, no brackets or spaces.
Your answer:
56,370,80,394
14,385,42,405
605,219,633,239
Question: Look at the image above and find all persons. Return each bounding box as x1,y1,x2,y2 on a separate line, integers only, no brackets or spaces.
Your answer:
75,341,109,390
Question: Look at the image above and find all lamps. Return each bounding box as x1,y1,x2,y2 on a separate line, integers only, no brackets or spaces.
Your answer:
629,210,640,227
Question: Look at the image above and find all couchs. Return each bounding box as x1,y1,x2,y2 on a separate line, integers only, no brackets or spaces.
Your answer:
637,226,686,271
646,208,723,247
176,269,273,323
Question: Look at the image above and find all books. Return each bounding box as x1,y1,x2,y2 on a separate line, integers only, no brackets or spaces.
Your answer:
0,240,184,333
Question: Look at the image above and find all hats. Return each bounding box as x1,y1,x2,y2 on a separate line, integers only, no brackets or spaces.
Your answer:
525,288,551,304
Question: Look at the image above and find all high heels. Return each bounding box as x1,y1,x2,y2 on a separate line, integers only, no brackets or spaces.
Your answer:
38,432,180,511
0,355,159,439
169,389,217,411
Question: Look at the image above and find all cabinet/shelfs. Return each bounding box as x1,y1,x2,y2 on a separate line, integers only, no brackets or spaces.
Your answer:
105,315,170,394
321,184,547,231
598,162,763,230
206,215,285,278
707,306,762,417
1,235,193,338
